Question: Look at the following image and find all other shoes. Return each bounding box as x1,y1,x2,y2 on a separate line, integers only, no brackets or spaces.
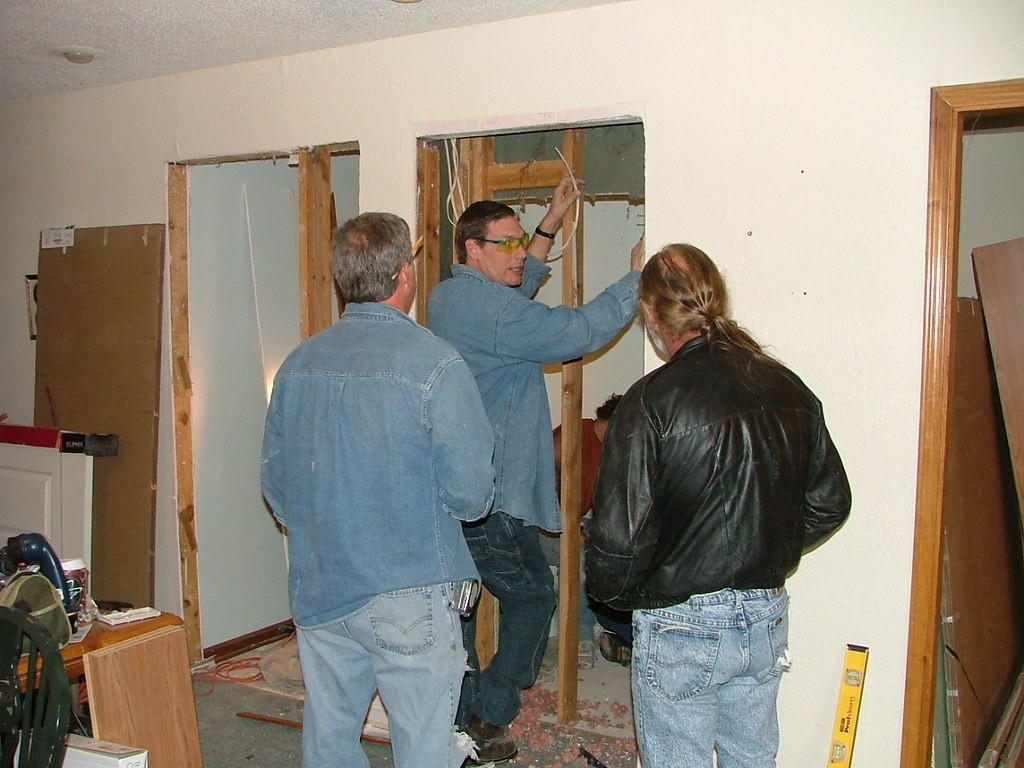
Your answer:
577,639,595,668
457,717,517,762
598,631,631,667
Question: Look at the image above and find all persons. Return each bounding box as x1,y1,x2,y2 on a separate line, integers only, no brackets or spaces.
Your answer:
428,169,645,763
259,212,497,768
583,245,852,768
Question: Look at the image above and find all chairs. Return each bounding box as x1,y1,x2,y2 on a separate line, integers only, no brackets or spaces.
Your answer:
0,605,71,768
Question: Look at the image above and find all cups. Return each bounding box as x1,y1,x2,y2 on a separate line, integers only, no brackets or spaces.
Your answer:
60,558,86,624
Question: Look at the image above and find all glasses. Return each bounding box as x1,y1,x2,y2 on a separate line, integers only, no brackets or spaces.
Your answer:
472,232,530,254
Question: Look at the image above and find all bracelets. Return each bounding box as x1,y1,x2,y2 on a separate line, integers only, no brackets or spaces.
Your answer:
534,224,556,240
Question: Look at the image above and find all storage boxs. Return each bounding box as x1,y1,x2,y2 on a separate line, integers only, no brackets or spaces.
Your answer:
13,728,148,768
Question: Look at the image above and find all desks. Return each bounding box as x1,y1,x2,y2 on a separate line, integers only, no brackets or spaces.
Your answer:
16,601,184,695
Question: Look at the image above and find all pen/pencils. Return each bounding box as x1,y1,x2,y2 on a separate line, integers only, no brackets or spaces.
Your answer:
392,235,423,280
